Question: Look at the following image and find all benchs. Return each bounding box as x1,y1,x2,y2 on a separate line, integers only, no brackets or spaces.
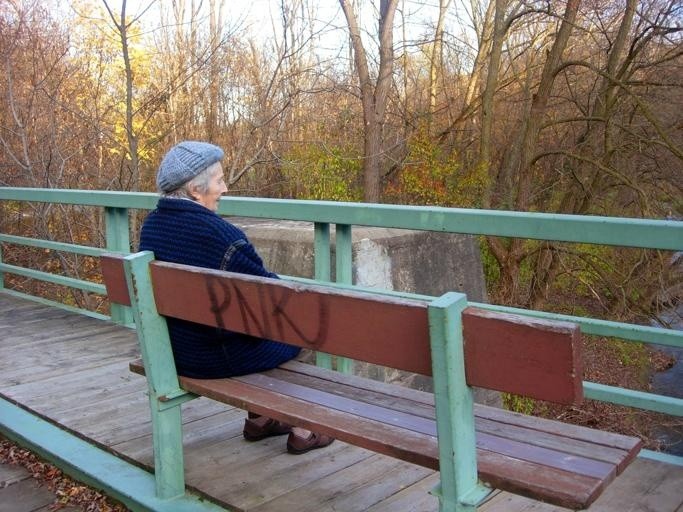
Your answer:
95,250,647,512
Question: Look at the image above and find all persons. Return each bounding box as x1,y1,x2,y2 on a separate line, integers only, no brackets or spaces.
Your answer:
138,140,336,455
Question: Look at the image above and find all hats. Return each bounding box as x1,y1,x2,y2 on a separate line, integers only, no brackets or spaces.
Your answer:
157,141,224,194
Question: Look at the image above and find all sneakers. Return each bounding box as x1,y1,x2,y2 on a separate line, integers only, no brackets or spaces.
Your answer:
244,418,294,442
287,428,334,454
